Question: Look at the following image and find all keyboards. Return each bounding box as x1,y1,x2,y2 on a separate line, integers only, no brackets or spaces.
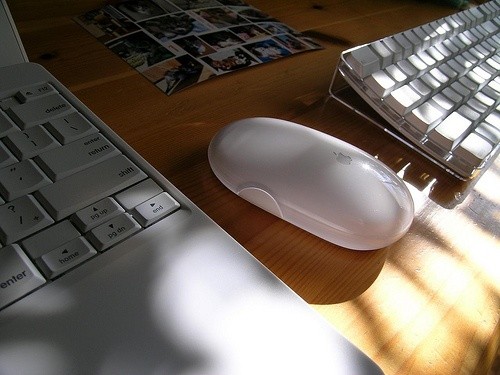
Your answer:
328,0,500,182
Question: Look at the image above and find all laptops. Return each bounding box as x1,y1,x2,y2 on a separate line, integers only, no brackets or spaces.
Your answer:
0,0,386,375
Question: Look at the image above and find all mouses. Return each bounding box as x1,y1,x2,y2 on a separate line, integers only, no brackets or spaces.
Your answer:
207,116,415,252
